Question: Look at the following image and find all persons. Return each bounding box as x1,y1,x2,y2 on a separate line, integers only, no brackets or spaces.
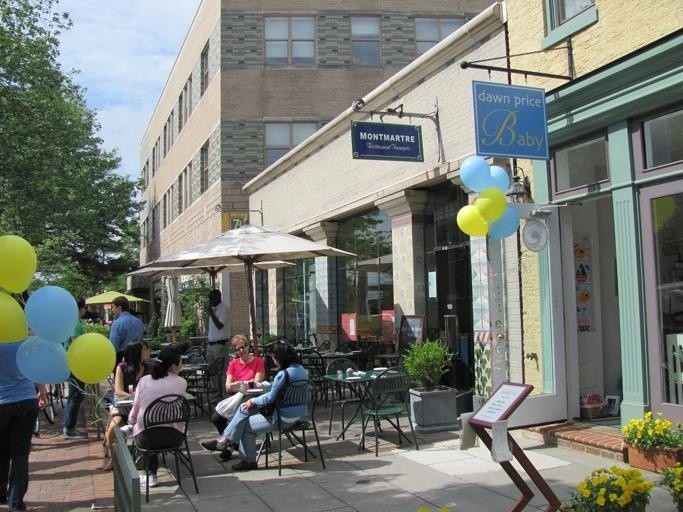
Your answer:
212,334,266,459
0,335,49,511
104,339,155,459
62,296,88,440
201,340,309,470
127,345,187,488
109,295,145,381
206,288,231,414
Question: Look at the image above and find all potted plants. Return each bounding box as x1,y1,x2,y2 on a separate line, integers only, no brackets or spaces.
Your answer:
402,338,459,434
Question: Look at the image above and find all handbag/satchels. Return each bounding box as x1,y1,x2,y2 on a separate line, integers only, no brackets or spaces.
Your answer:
258,403,276,417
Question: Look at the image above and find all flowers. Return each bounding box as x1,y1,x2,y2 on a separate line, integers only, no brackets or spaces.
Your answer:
659,462,683,506
623,410,683,452
567,466,654,512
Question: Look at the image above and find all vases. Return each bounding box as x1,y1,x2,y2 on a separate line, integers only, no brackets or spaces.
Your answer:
626,446,683,473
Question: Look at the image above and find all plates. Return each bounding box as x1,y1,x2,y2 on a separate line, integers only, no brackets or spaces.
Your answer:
116,400,134,404
374,367,388,371
246,389,262,392
345,377,361,380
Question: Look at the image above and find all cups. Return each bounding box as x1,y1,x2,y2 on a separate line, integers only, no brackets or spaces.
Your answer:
248,380,254,389
346,368,353,377
337,371,343,379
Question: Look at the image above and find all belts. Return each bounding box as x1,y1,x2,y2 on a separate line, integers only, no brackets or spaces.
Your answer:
208,339,228,345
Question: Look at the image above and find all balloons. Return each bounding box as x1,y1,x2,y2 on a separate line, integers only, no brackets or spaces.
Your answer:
24,286,79,343
16,336,70,384
0,234,37,294
0,291,29,344
456,154,521,240
64,331,117,384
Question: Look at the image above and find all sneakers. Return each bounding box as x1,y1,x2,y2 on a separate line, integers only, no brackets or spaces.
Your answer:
201,440,257,470
64,431,87,439
148,476,157,487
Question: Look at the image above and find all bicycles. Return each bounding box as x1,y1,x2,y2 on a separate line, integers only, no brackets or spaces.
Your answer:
45,382,66,415
33,394,54,436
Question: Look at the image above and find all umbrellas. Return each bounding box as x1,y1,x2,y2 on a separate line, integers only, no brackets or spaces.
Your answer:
138,219,357,358
164,275,183,343
123,260,296,290
84,290,151,323
